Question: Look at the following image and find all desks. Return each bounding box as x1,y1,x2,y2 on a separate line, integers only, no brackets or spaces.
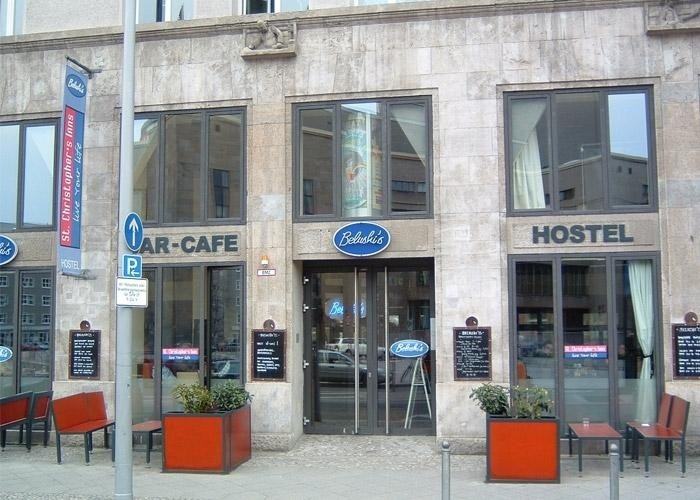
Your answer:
111,420,165,468
567,421,624,479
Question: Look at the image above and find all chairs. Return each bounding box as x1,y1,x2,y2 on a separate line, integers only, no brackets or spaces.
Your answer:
624,391,691,478
0,390,116,466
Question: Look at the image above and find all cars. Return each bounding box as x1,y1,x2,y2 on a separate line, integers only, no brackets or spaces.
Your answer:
21,340,50,351
312,348,395,386
210,342,242,379
326,335,387,360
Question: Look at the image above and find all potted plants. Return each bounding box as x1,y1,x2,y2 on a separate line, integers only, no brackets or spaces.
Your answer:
468,380,562,484
160,378,258,476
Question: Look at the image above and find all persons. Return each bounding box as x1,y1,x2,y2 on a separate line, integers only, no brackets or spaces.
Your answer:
660,1,681,28
618,343,628,359
247,19,285,49
217,341,226,353
534,341,554,378
